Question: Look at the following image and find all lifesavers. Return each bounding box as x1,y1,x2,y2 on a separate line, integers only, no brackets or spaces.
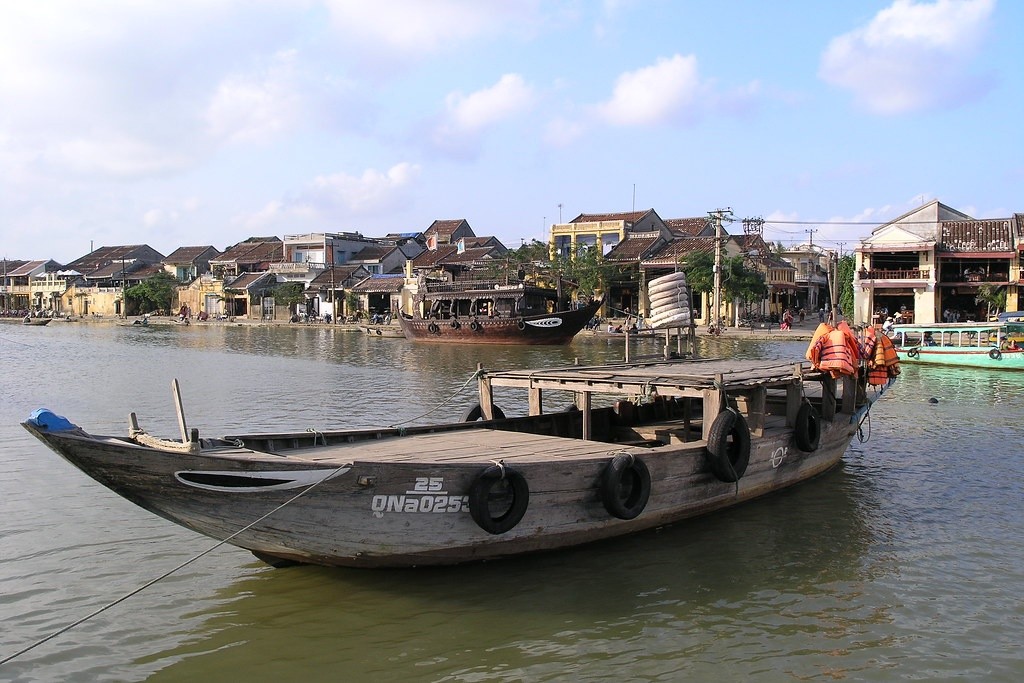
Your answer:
601,452,652,521
376,330,381,335
470,322,479,331
459,402,507,422
907,348,917,357
517,321,526,331
427,323,436,334
450,321,458,330
707,409,752,484
989,349,1000,359
469,465,530,536
564,404,579,411
794,403,822,453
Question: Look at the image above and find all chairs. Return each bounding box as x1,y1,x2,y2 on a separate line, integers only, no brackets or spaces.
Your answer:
911,267,919,279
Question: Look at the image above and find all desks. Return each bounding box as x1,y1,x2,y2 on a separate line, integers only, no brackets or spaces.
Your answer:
901,310,914,324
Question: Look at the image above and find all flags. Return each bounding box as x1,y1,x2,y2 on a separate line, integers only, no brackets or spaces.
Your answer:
425,233,438,250
457,238,466,254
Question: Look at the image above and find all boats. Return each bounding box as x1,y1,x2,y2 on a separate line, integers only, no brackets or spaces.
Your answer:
23,318,53,326
889,322,1024,370
359,325,406,338
394,266,606,346
18,359,901,572
116,320,149,327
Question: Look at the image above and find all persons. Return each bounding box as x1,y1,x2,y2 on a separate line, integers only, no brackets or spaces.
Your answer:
582,306,655,335
288,306,406,325
0,305,59,318
479,306,501,320
133,304,239,324
858,263,1024,350
692,301,842,336
